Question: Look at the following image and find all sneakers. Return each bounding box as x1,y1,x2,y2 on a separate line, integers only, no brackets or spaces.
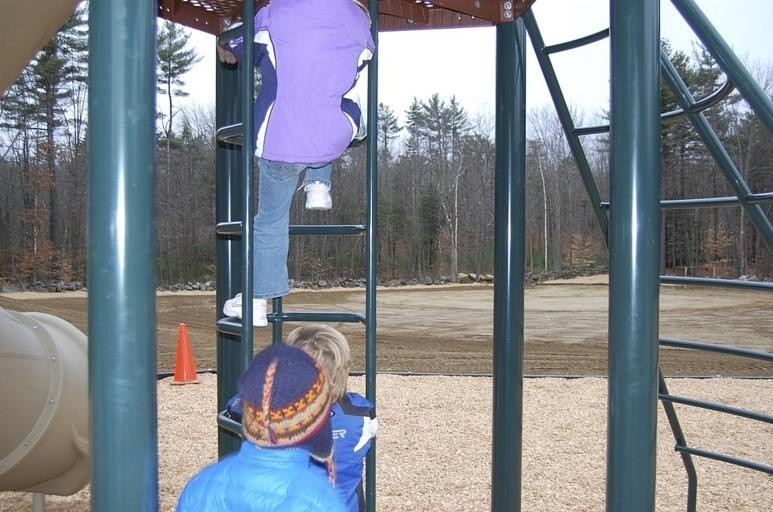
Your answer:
304,184,332,210
223,293,268,327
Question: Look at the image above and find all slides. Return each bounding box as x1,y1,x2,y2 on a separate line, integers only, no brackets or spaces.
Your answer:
1,306,91,495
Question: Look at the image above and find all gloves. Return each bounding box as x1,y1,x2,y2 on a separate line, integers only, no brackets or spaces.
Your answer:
217,41,237,64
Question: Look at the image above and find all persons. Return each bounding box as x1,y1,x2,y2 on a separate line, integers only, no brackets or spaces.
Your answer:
174,339,350,512
213,0,376,329
223,322,379,512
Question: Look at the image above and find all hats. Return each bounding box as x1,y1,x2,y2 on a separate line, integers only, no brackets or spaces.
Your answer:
240,344,335,486
